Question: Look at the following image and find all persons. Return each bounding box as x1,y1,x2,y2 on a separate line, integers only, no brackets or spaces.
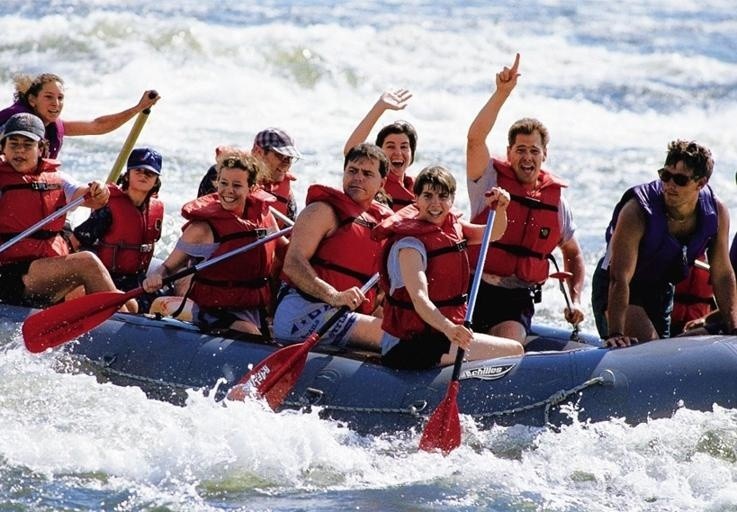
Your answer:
379,166,524,364
144,150,286,336
1,71,161,155
71,148,165,310
201,128,297,238
466,53,585,344
0,111,139,316
274,145,396,354
345,86,418,212
590,141,736,350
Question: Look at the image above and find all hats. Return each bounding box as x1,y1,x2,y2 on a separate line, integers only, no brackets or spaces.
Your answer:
127,149,162,175
0,113,45,142
255,128,302,160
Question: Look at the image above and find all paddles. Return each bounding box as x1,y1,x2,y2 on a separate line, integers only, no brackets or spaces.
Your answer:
226,271,382,411
22,225,294,354
418,190,500,455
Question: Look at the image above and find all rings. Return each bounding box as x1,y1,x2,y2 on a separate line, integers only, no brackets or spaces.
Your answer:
352,299,357,306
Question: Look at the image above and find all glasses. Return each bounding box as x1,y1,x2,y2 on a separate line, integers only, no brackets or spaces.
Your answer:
269,150,293,160
658,169,703,186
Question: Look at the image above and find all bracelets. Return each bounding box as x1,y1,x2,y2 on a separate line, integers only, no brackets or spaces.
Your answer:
330,292,341,308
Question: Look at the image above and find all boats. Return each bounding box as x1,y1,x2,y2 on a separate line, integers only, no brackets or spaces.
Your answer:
0,255,737,454
